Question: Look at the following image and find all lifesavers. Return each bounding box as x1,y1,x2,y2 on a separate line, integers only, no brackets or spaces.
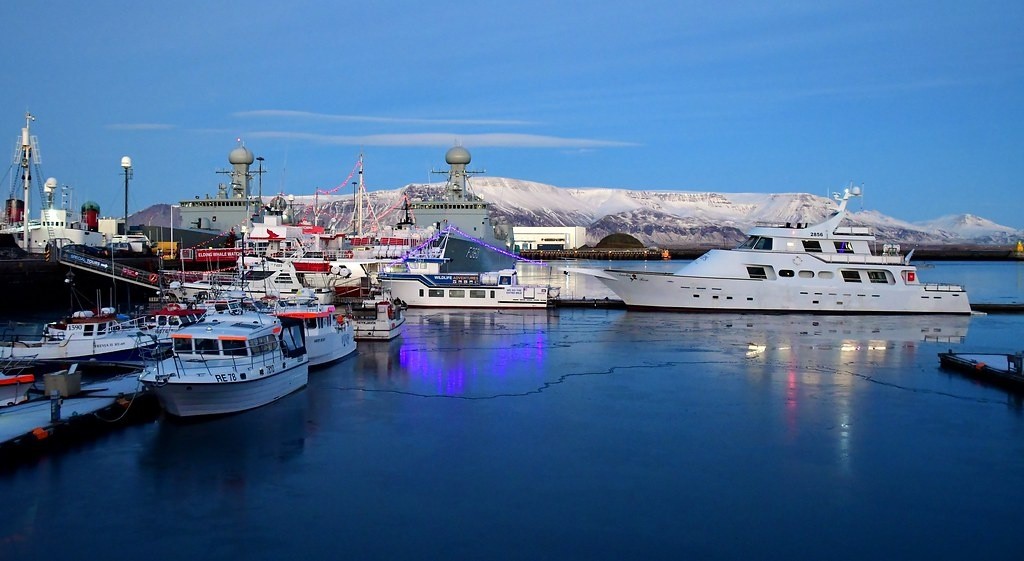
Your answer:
169,303,180,312
388,306,393,318
149,274,158,283
345,250,354,259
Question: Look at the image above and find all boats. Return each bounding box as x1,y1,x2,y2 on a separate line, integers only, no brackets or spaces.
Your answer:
0,110,973,448
599,310,970,369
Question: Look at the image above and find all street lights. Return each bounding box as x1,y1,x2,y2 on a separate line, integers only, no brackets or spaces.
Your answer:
121,156,131,235
43,178,58,209
